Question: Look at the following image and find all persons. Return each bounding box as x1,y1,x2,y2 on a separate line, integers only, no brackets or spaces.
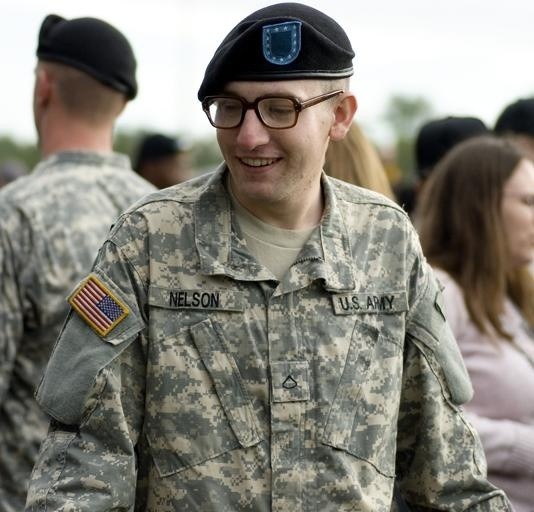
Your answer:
323,120,398,203
1,14,159,512
412,136,534,511
24,2,517,511
493,97,534,161
132,134,185,189
415,116,487,190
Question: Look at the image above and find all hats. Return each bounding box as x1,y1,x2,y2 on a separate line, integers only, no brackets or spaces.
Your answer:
493,94,534,134
412,118,488,177
198,1,356,102
35,13,138,101
136,134,179,163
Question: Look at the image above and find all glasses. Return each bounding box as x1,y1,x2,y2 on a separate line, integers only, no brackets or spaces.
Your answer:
200,92,343,131
